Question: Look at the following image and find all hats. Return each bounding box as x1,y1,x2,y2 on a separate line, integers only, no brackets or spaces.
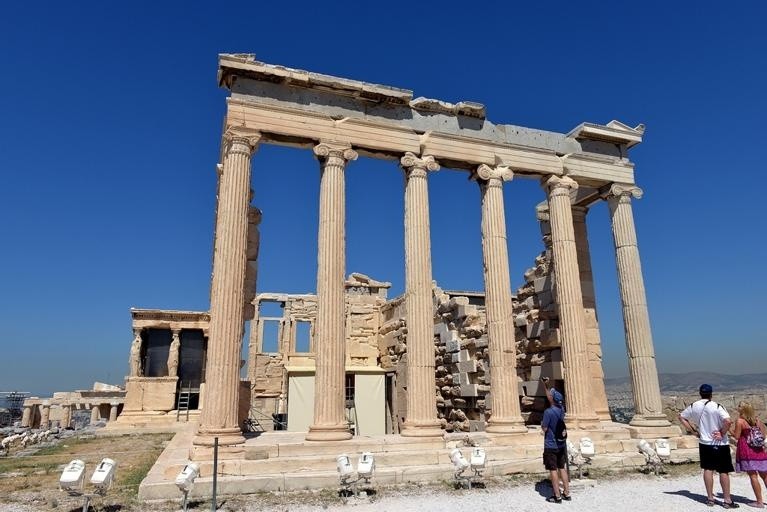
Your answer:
550,387,563,402
700,384,713,394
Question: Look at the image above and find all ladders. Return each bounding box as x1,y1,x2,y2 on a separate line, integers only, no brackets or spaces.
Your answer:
177,380,191,422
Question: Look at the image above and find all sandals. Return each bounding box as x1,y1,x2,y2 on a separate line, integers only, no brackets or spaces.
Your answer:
561,493,571,501
546,496,562,504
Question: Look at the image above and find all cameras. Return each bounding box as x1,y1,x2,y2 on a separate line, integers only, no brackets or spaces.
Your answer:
542,377,548,382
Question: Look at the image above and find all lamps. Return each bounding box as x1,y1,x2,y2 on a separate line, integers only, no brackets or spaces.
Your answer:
338,453,374,499
174,463,201,512
60,455,117,512
639,437,670,477
566,437,594,480
449,447,486,489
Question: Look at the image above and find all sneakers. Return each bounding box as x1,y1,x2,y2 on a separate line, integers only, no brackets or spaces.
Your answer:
723,499,740,509
748,502,764,508
707,498,716,507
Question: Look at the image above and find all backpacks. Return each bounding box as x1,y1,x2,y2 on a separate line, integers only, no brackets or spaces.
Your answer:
746,425,764,449
550,407,567,442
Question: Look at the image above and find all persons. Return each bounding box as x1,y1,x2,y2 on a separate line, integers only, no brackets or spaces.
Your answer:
540,376,570,503
677,383,739,508
727,400,766,508
